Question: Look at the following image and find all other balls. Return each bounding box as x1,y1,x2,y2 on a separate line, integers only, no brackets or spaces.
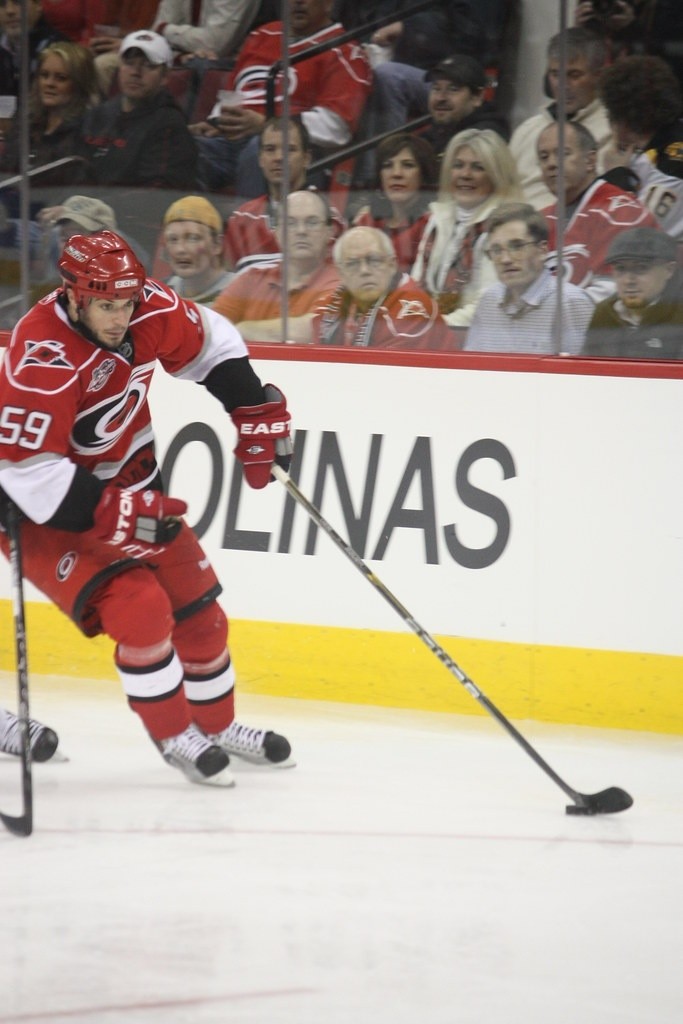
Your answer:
565,805,598,816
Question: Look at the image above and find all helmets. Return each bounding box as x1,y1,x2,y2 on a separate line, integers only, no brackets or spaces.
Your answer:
58,230,146,309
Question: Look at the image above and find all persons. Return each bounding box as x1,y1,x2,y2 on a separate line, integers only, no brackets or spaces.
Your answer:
0,0,683,360
0,231,297,788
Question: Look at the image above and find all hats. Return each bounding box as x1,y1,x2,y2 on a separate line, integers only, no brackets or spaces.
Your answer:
119,29,173,69
48,195,118,234
604,229,677,264
424,54,486,88
163,195,223,234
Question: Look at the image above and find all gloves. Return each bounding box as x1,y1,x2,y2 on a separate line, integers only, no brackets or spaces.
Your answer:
230,383,292,489
78,486,187,560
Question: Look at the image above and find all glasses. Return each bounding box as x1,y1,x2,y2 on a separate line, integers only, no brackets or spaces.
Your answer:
483,240,540,263
340,255,394,273
280,216,327,232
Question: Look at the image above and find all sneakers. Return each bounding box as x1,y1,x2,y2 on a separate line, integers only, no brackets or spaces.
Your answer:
0,708,70,762
204,719,296,770
154,724,237,787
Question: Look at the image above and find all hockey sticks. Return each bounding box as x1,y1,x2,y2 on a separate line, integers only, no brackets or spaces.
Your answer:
268,460,632,816
0,496,34,839
